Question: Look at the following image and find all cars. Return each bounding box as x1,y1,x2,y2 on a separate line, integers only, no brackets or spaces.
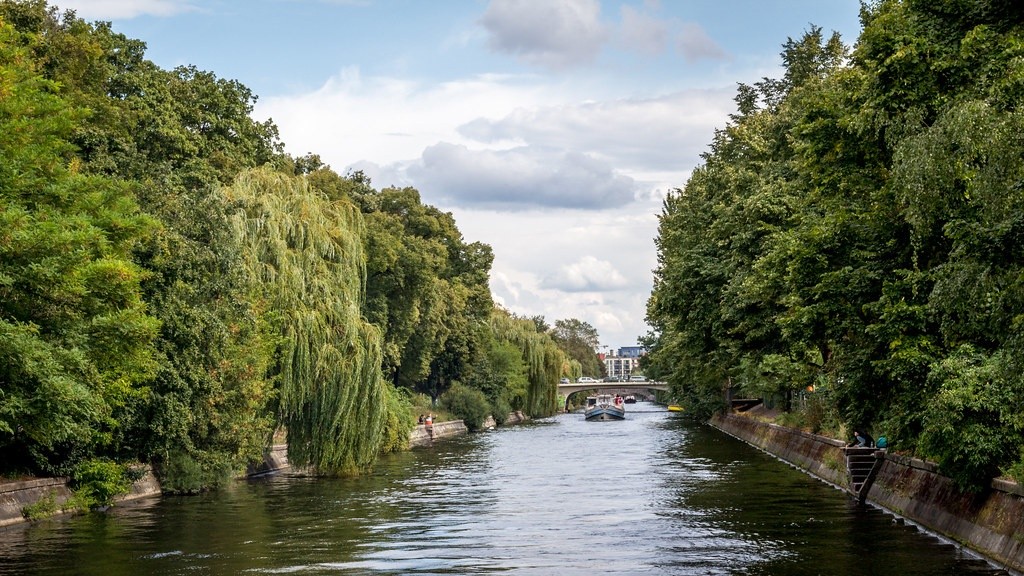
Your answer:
603,376,625,382
576,376,598,383
561,378,570,383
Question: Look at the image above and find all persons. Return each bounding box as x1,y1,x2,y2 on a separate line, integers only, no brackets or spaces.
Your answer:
846,430,875,448
419,414,424,425
425,416,432,424
628,373,631,382
618,375,627,382
613,394,623,406
876,436,888,448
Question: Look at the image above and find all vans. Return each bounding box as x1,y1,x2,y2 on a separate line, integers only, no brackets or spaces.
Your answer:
629,375,647,382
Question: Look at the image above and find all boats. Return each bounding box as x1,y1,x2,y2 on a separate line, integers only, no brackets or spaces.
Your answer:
624,395,637,404
584,392,626,421
667,404,684,412
